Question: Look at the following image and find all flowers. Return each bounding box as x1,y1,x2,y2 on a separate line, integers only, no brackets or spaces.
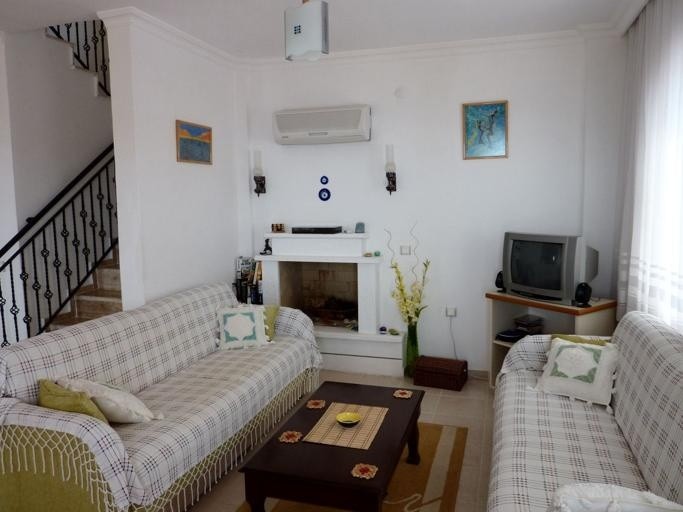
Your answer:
389,259,430,326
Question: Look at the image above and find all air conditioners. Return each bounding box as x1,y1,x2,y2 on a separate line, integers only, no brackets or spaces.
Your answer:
271,104,370,144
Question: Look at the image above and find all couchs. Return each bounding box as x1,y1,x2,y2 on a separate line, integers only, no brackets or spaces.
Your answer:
0,280,321,512
486,309,682,512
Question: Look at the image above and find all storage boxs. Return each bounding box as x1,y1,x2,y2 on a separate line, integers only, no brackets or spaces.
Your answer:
414,355,467,390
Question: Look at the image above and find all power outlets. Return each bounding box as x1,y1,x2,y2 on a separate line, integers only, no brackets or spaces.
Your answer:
445,305,457,317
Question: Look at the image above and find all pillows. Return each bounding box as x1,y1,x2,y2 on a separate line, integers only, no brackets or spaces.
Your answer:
215,304,275,351
537,333,618,413
231,303,277,341
545,482,683,512
36,378,107,423
56,378,162,422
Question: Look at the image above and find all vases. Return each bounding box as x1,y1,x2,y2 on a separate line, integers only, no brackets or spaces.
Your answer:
407,317,417,377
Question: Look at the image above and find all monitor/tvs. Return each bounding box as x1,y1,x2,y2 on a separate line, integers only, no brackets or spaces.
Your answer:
503,232,599,307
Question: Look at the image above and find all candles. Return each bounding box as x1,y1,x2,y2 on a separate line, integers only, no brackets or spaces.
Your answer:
253,150,261,168
385,144,393,164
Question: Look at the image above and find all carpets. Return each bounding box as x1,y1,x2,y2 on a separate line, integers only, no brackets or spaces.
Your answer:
235,421,467,512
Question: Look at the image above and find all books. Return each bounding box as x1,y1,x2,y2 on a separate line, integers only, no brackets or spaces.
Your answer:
228,254,262,305
514,315,543,326
517,326,543,337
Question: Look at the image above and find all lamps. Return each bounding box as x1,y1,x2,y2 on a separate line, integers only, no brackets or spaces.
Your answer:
284,0,328,61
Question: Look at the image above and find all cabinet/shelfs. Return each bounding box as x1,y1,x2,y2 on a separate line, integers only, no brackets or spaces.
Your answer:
484,291,616,390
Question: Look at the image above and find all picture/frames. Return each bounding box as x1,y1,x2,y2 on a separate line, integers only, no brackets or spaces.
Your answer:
462,99,508,160
175,118,211,165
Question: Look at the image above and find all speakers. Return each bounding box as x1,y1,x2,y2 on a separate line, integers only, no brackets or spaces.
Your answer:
575,282,592,308
495,271,503,293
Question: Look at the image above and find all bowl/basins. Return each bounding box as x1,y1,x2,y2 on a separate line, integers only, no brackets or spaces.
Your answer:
336,411,362,426
